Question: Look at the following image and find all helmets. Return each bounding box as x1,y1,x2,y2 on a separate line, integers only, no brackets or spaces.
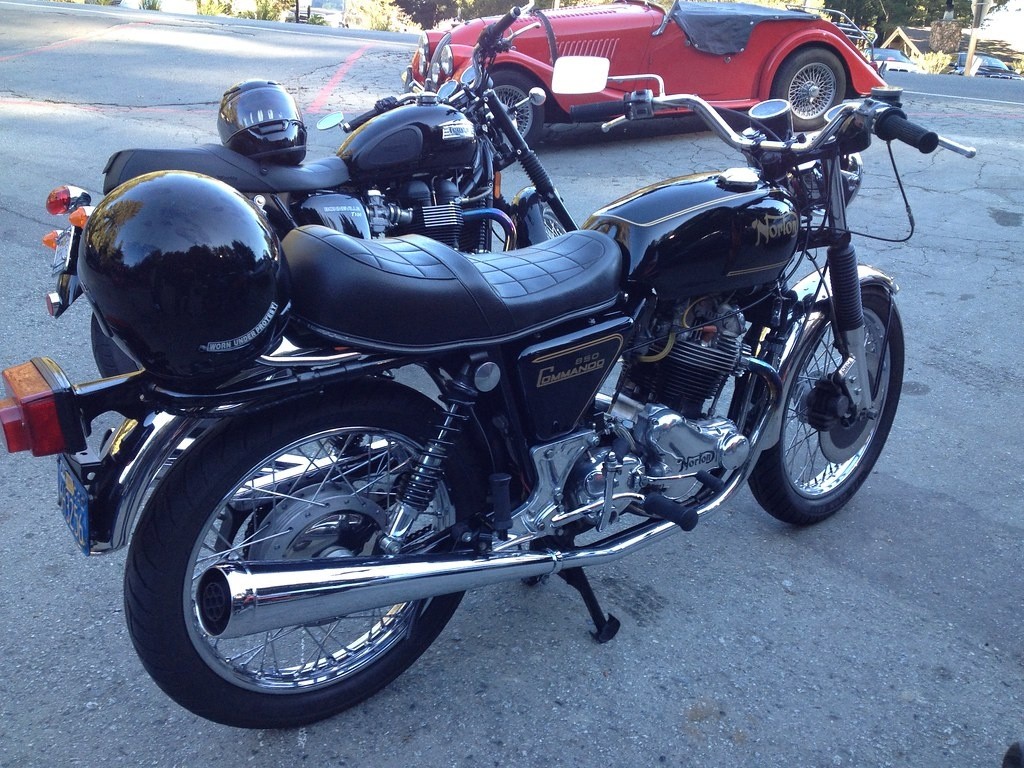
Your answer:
75,169,296,389
216,79,307,167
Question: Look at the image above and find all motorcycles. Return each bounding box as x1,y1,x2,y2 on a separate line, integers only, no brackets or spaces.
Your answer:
43,7,578,378
1,69,982,736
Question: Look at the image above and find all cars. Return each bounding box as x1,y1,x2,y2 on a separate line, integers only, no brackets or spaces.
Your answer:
938,53,1021,81
863,44,924,75
402,1,893,149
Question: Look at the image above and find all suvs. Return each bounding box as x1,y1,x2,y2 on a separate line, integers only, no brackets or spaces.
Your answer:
285,0,344,27
